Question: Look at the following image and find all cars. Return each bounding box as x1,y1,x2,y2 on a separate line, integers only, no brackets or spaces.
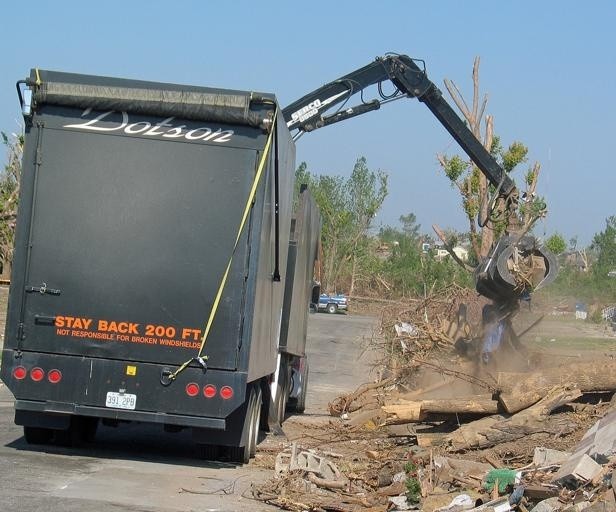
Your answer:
318,293,348,314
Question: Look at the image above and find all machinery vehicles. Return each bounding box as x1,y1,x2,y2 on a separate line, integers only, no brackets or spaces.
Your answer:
0,51,561,464
